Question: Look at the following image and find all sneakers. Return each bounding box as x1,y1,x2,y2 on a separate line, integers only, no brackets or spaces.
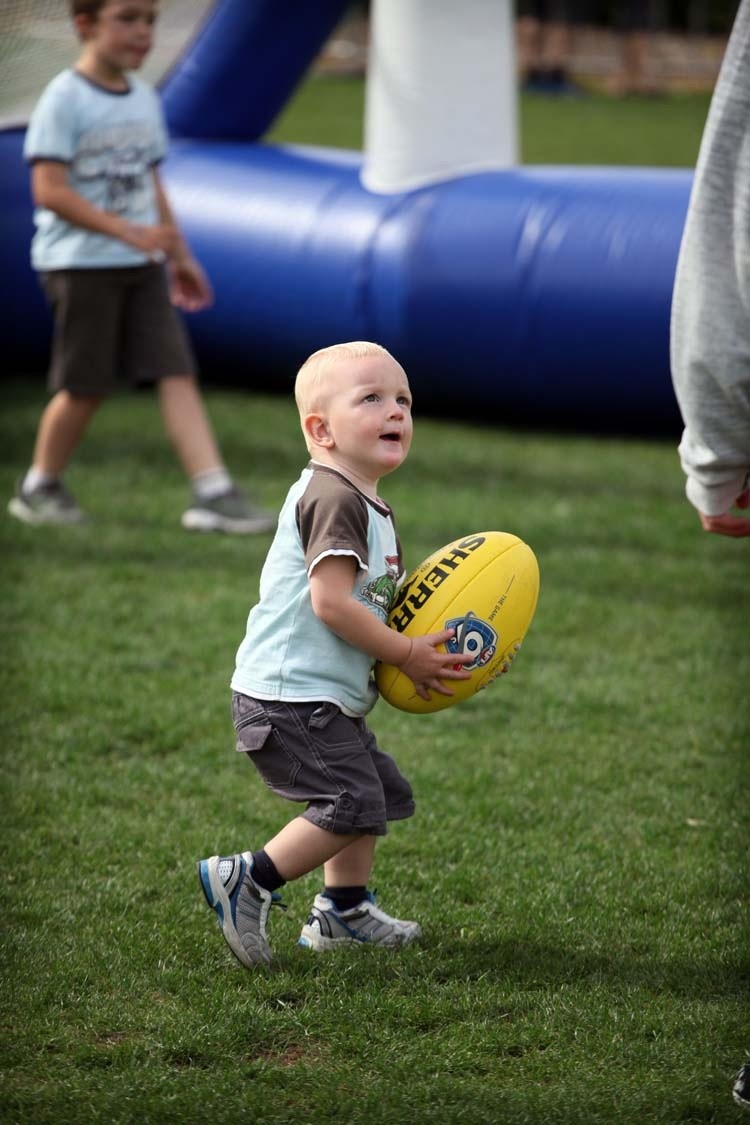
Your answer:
195,849,288,971
296,891,423,949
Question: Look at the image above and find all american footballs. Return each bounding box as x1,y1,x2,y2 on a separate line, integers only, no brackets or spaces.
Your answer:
376,531,543,719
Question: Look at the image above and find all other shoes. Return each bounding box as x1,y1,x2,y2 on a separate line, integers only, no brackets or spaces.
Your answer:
180,487,275,535
7,471,86,524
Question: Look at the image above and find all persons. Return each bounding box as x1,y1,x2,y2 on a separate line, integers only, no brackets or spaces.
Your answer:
667,1,750,538
197,341,519,970
6,0,277,541
